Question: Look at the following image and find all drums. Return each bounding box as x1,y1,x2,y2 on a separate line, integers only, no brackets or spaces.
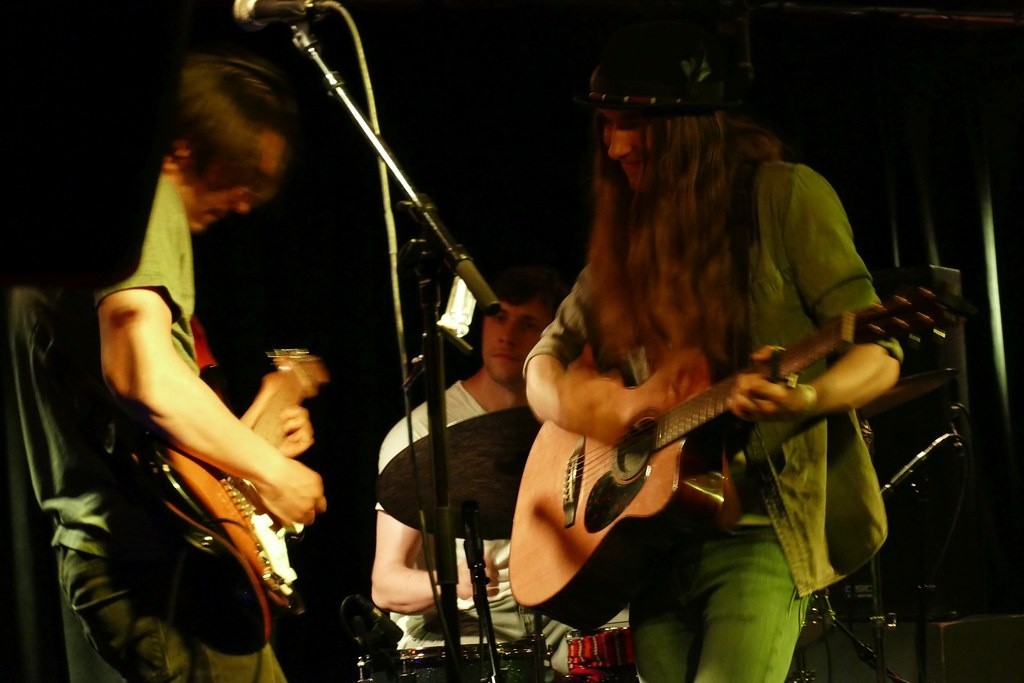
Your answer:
357,639,542,683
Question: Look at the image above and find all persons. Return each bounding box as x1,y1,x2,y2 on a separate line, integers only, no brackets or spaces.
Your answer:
368,249,630,683
9,52,329,683
521,1,909,683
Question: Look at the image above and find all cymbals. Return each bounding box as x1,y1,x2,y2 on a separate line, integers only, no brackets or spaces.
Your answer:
373,404,541,542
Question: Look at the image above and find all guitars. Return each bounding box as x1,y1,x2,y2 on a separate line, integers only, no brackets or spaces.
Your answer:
508,284,966,633
166,346,333,621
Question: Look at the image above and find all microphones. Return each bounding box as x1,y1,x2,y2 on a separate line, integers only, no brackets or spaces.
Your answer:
235,0,328,27
355,594,402,643
879,450,927,499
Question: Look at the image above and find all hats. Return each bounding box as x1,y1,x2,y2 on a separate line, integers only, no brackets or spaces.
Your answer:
571,21,753,111
